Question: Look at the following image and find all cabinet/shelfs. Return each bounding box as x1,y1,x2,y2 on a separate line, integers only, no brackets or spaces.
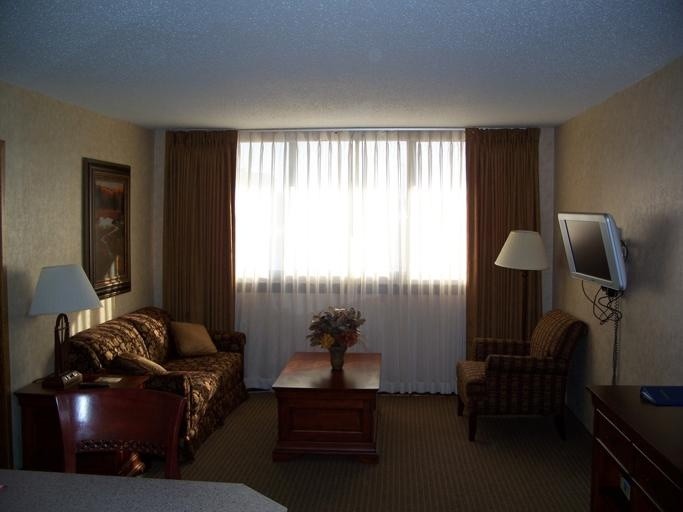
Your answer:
13,374,150,476
586,384,683,512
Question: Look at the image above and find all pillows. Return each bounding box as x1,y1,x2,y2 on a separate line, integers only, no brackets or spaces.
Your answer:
114,352,167,373
169,314,217,357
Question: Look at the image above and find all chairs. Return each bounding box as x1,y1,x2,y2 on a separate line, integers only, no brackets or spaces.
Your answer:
55,389,188,480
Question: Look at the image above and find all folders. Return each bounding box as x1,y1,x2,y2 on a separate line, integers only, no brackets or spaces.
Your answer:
640,383,683,406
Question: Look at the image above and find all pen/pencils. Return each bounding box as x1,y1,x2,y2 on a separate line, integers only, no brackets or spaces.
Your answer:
78,382,111,389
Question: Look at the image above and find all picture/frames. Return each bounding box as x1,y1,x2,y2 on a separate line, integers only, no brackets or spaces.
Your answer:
82,157,132,300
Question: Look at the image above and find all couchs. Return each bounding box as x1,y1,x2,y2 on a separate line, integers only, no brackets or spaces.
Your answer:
65,307,249,457
456,308,583,441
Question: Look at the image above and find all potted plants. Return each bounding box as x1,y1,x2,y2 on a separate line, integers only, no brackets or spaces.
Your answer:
306,305,366,370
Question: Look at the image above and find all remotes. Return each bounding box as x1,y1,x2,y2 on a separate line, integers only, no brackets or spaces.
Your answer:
77,381,112,389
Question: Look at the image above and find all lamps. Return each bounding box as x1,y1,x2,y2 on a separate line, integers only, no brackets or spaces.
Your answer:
28,264,104,377
494,230,549,340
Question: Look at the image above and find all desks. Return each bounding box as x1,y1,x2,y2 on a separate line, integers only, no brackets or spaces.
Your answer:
0,469,288,512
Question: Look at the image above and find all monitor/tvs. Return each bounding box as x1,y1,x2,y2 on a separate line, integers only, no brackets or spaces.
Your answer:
556,211,628,292
